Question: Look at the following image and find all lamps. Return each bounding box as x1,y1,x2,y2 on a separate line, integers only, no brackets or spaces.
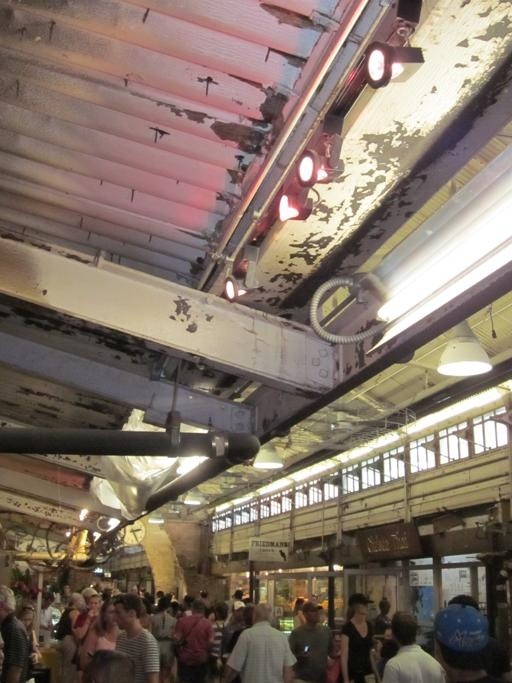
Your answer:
434,317,496,378
223,0,427,302
146,512,167,524
252,440,286,468
184,486,204,506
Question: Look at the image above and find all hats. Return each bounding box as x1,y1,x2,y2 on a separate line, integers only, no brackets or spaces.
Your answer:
303,601,322,611
348,593,374,604
424,604,488,650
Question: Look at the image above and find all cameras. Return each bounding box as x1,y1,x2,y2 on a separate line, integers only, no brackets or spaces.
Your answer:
303,644,312,654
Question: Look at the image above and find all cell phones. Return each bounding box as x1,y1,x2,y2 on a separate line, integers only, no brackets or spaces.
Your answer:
87,651,94,656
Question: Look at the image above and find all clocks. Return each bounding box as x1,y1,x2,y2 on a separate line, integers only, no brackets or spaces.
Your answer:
123,519,146,545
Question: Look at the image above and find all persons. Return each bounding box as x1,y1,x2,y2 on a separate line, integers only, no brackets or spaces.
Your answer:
1,578,512,683
